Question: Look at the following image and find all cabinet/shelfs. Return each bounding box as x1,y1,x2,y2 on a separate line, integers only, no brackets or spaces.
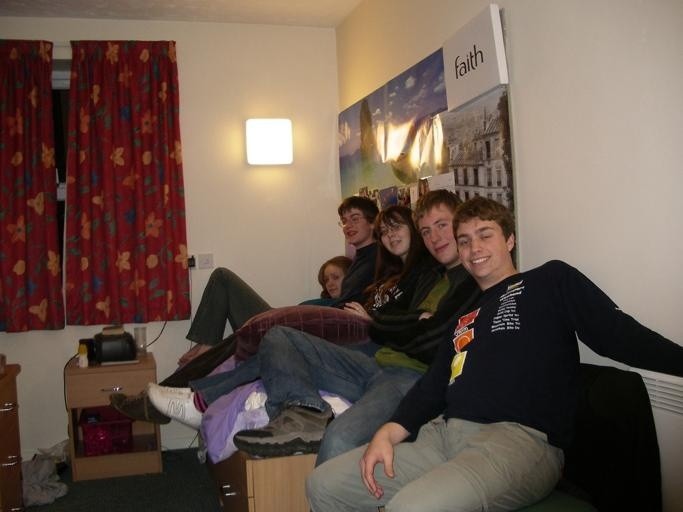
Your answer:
0,364,26,512
214,449,318,512
64,353,163,482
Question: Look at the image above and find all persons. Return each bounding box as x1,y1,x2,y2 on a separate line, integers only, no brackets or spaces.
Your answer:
109,195,415,431
306,198,683,512
233,189,482,470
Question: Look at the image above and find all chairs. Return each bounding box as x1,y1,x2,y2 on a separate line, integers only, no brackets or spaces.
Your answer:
516,363,662,512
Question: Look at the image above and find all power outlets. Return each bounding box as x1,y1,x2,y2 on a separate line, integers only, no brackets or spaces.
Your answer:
187,254,198,269
198,253,214,269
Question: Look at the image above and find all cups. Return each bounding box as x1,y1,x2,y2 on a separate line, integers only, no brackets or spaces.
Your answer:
134,327,147,358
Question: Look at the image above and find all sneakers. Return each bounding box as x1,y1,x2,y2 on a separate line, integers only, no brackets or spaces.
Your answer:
178,344,207,365
233,404,336,458
110,382,205,428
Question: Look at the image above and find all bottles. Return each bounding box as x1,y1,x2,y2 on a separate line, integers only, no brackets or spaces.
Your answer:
79,344,89,369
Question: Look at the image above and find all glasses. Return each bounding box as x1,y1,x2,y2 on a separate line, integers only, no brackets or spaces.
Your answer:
338,216,362,227
380,222,403,236
